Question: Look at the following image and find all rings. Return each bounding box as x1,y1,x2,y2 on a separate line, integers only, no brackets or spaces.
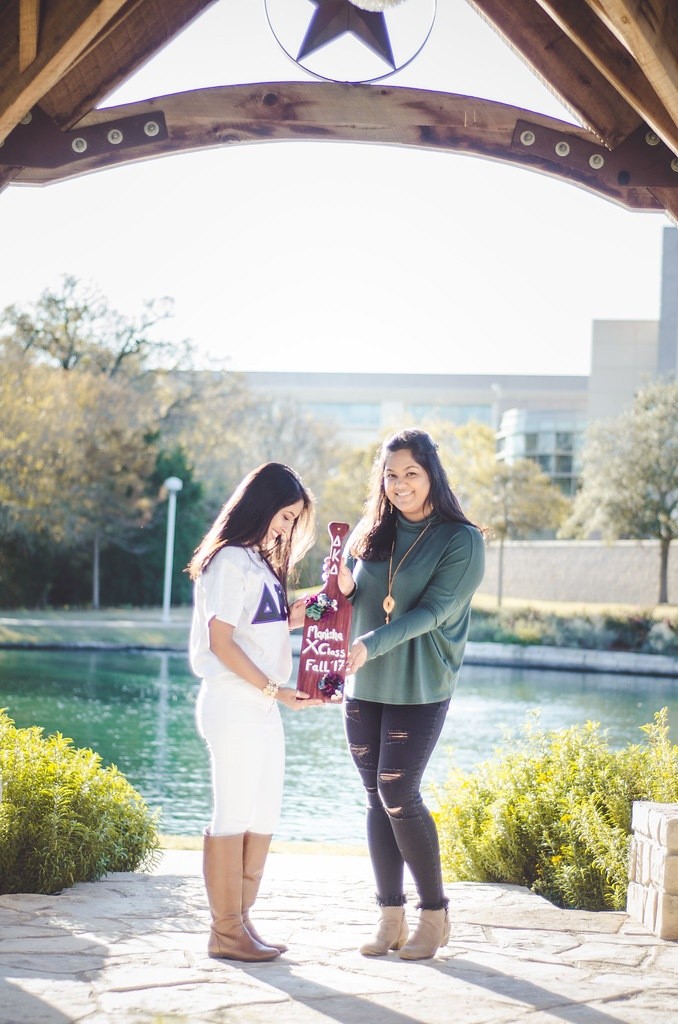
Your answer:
296,689,300,697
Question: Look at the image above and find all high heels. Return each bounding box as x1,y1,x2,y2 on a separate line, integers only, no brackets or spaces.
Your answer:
361,905,409,958
401,909,450,960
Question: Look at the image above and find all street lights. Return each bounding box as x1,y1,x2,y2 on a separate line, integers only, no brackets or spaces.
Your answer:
164,476,184,618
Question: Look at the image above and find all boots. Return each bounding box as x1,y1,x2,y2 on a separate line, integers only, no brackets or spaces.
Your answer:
202,826,280,961
241,832,288,954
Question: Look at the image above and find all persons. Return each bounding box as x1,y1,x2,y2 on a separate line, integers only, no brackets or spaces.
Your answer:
322,429,486,961
183,464,323,963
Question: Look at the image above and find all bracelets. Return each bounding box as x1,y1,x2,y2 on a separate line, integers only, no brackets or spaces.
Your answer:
263,680,279,697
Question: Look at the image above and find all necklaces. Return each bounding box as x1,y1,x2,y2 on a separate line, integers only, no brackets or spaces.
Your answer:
382,520,431,625
